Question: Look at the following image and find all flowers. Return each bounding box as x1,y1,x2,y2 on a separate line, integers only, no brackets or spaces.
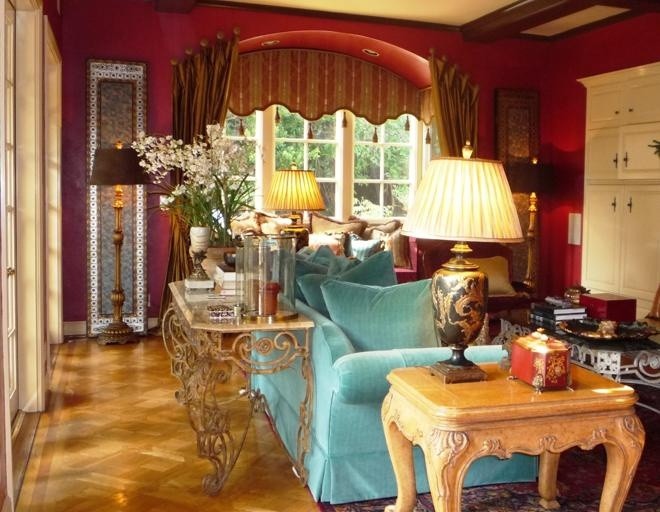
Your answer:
132,122,255,248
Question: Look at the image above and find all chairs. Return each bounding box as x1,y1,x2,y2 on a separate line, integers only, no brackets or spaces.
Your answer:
238,204,310,251
421,240,533,333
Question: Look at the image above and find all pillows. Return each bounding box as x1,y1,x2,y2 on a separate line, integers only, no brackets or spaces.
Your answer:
269,244,442,352
230,211,293,239
450,255,517,297
310,212,412,270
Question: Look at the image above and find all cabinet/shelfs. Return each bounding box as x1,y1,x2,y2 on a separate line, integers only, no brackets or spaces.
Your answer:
576,61,660,300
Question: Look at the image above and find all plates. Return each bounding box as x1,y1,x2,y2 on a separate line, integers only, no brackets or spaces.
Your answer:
559,317,658,346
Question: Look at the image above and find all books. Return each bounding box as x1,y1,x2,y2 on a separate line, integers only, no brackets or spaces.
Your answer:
212,264,261,296
529,301,588,327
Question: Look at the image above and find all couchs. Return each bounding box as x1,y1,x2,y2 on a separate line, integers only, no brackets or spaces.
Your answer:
394,236,423,284
250,286,540,507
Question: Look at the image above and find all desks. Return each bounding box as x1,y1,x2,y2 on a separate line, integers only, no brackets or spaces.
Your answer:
160,279,315,498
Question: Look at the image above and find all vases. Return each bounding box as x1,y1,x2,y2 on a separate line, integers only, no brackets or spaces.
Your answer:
190,226,210,253
208,246,237,294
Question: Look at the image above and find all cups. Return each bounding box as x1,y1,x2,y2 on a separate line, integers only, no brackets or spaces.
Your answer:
250,280,279,316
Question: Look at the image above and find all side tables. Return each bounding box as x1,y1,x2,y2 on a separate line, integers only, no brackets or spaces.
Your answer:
380,361,647,511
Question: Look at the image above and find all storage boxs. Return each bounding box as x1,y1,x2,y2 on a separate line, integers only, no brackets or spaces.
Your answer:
580,292,637,325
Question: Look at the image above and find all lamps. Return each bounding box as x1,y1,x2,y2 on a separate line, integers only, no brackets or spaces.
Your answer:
88,140,152,346
261,160,327,252
398,138,526,385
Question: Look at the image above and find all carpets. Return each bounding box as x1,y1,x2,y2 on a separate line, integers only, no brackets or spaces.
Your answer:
238,358,660,512
